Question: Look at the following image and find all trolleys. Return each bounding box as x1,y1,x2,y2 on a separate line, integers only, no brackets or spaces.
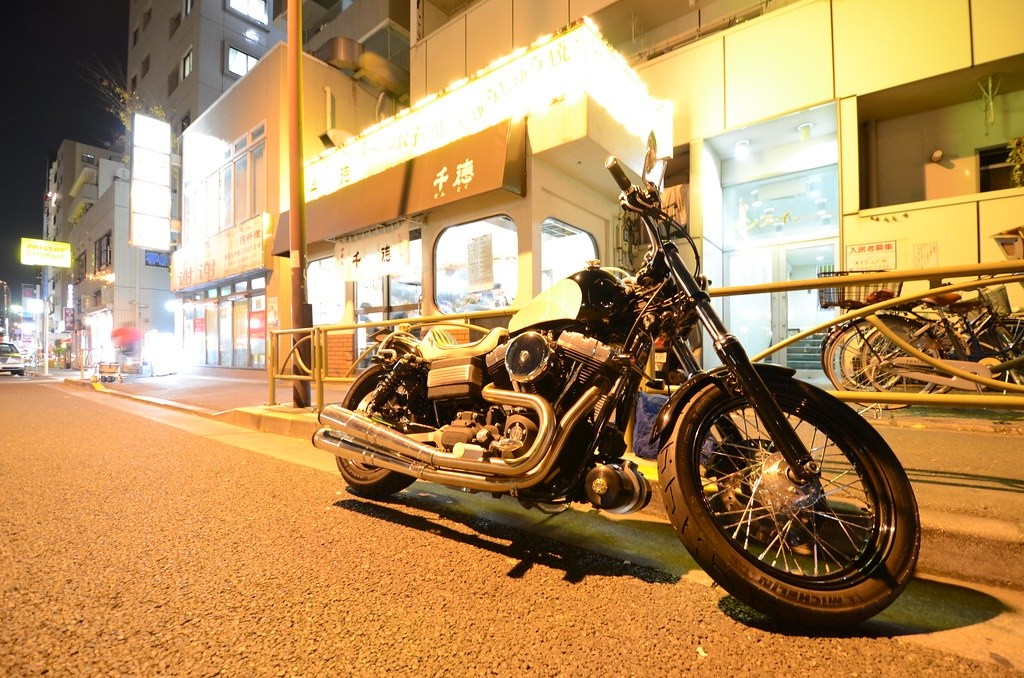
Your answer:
90,344,125,384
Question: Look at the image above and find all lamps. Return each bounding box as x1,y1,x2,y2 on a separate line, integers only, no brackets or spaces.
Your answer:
732,139,749,164
799,122,813,142
319,128,355,149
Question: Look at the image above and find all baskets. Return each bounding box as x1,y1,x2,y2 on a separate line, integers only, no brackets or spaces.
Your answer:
962,286,1012,326
817,270,905,310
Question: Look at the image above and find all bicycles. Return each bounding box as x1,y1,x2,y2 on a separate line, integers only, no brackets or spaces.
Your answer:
814,261,1024,413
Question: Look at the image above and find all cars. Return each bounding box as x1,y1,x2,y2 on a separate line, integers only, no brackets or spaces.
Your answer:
0,341,28,377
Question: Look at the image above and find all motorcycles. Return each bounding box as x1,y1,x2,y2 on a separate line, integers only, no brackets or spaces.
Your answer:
311,131,924,630
